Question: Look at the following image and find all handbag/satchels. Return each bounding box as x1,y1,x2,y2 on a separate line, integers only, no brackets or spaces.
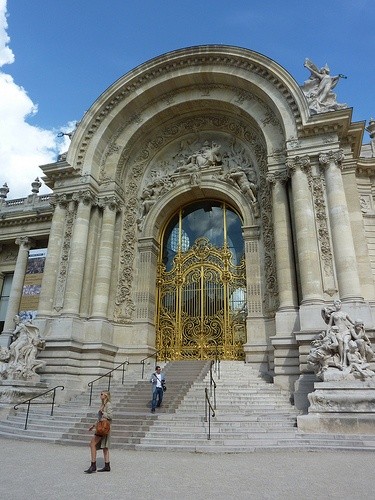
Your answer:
94,418,110,436
163,385,166,392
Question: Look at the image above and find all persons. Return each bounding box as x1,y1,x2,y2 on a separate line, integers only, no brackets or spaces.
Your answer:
149,365,165,414
140,142,256,203
307,298,375,381
83,390,111,473
303,64,341,104
0,314,46,379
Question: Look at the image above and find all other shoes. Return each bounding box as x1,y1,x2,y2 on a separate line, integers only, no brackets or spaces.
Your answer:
157,404,160,407
152,408,155,412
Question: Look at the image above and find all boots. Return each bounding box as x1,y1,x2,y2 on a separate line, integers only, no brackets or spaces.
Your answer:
84,461,96,473
97,462,110,472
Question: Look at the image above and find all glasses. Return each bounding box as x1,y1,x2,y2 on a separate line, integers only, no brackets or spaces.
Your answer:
100,394,105,395
